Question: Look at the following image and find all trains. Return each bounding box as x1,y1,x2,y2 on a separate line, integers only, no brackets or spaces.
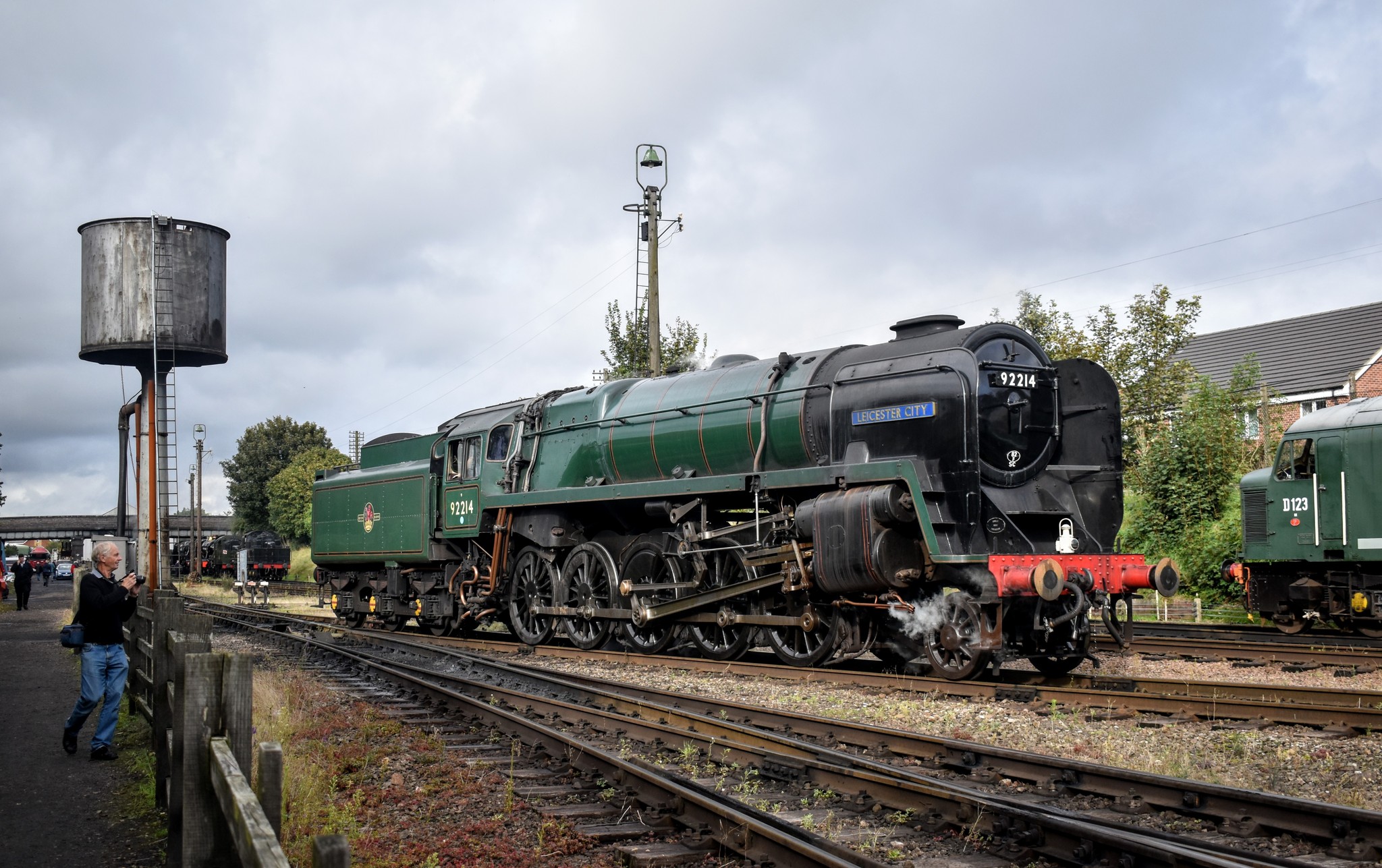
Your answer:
171,530,292,582
1220,395,1382,636
309,314,1180,681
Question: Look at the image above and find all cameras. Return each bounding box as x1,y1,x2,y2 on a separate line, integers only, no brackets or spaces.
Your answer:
127,573,146,585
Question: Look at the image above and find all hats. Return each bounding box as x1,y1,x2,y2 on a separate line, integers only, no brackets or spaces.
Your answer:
18,554,24,558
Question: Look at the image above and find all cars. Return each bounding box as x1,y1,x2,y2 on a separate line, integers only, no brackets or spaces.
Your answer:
55,560,72,580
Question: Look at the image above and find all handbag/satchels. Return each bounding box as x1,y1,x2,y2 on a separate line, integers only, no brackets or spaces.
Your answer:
59,624,84,649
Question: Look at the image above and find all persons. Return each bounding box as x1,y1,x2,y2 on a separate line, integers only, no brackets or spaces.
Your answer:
25,556,86,587
175,558,198,578
62,541,141,761
10,554,34,611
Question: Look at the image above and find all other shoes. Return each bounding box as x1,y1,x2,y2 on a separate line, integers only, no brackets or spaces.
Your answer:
16,607,22,611
46,585,49,587
90,746,119,761
63,729,78,755
54,579,56,580
51,579,53,581
43,582,46,586
38,579,40,581
23,605,28,610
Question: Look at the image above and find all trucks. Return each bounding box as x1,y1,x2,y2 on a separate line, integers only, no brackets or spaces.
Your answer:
29,546,50,569
